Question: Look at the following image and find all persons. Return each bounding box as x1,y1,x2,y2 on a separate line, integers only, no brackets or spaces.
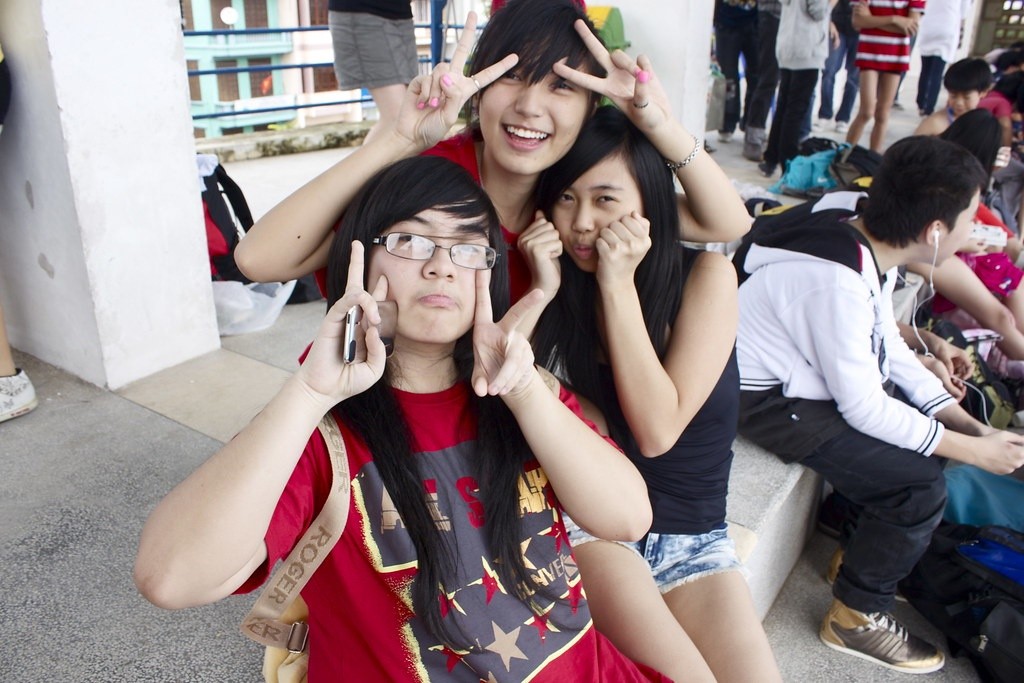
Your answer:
496,103,784,683
235,0,754,297
0,44,38,424
326,0,421,147
133,154,680,683
704,0,1024,676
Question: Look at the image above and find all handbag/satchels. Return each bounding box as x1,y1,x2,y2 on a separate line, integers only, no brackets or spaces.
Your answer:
768,137,885,195
893,306,1015,431
897,518,1024,683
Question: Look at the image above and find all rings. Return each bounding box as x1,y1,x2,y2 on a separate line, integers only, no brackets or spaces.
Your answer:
633,99,650,108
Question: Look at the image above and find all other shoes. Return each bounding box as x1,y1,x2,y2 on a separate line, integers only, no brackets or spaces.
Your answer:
835,120,848,132
812,119,829,132
759,163,772,177
743,142,763,161
719,131,732,143
817,496,842,539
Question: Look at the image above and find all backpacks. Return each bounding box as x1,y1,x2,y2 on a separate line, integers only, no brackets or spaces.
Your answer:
240,363,561,683
196,151,254,282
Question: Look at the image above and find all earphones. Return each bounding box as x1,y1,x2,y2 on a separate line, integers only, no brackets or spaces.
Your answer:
934,230,940,247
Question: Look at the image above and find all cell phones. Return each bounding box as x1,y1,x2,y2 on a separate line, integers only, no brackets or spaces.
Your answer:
343,305,366,365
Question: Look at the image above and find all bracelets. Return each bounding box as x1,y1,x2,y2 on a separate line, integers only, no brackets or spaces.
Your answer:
665,134,701,168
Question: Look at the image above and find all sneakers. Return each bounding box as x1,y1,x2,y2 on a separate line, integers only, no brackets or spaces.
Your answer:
826,546,909,603
0,368,37,422
819,599,945,674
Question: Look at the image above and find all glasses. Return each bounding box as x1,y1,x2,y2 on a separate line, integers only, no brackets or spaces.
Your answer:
370,232,501,270
